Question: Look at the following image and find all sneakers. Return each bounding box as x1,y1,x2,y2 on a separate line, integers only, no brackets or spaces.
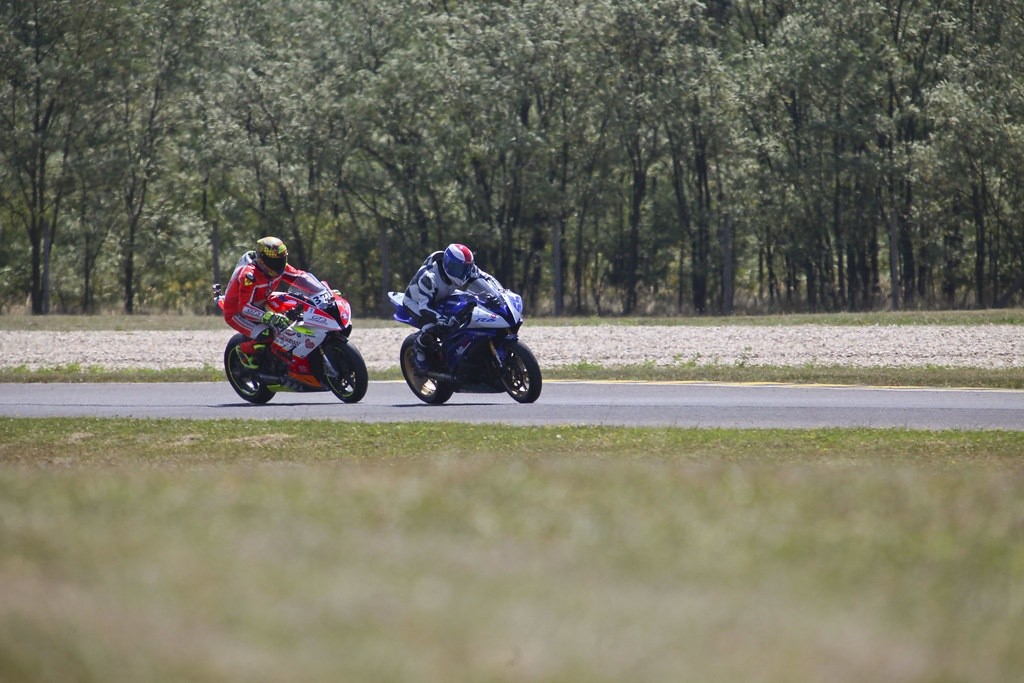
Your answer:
236,344,258,369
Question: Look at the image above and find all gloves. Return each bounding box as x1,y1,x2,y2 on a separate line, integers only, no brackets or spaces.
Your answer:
438,314,460,328
262,311,291,330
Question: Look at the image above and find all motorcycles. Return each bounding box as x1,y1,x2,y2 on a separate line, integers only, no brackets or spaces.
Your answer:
211,273,369,404
388,276,544,405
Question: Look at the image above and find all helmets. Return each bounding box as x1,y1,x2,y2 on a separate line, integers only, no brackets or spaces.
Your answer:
443,244,474,287
256,237,288,277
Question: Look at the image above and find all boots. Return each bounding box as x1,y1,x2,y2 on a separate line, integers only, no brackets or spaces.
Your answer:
412,331,432,373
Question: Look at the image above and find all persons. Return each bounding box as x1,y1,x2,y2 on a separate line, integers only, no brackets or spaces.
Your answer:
223,235,335,372
406,244,506,372
